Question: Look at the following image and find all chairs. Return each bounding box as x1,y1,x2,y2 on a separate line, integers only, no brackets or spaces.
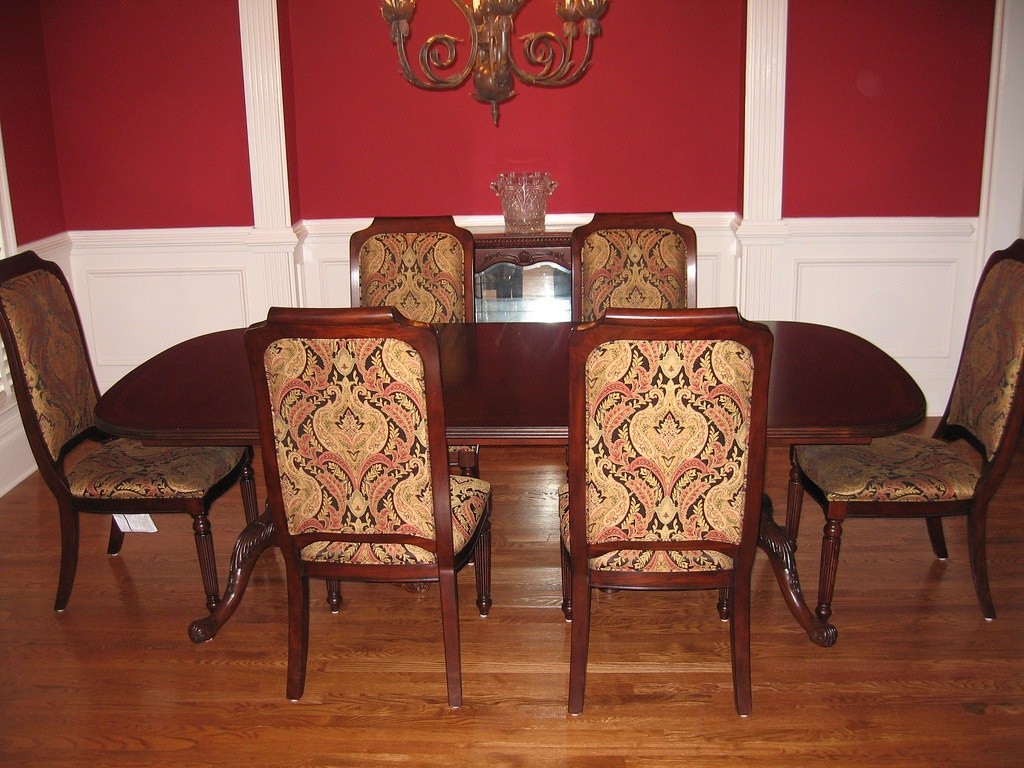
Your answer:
566,210,699,478
785,238,1024,622
243,306,493,708
557,306,775,716
349,215,473,479
0,251,259,614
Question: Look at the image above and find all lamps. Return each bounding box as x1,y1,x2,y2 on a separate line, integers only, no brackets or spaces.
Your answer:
378,0,608,124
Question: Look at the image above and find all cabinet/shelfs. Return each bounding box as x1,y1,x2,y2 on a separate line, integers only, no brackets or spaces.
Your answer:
472,232,572,322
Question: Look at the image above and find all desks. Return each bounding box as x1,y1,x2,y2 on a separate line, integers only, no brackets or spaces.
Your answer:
91,321,928,647
